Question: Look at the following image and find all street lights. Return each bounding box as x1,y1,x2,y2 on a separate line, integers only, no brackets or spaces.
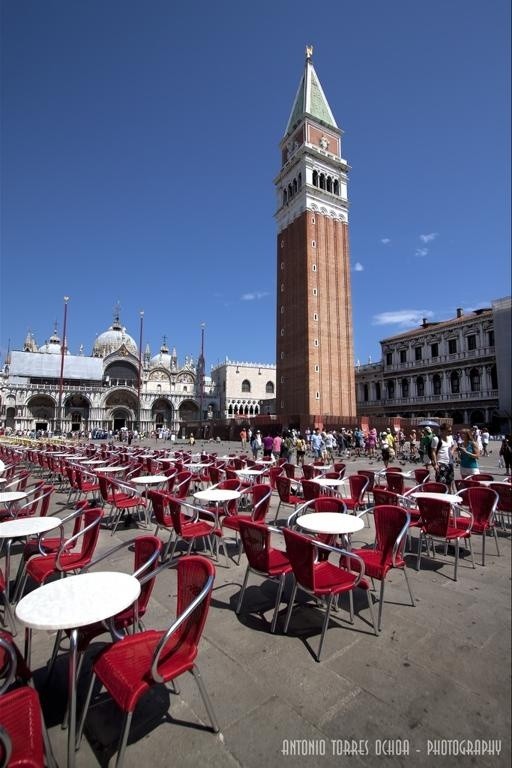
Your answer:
135,309,145,433
55,295,71,435
199,319,208,437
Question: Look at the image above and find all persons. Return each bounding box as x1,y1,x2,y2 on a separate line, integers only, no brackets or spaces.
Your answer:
238,421,512,478
1,425,195,448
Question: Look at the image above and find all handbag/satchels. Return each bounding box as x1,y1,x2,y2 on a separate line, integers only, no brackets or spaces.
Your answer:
428,435,442,458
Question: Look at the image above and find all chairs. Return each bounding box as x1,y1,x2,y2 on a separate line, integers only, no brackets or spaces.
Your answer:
1,425,512,768
